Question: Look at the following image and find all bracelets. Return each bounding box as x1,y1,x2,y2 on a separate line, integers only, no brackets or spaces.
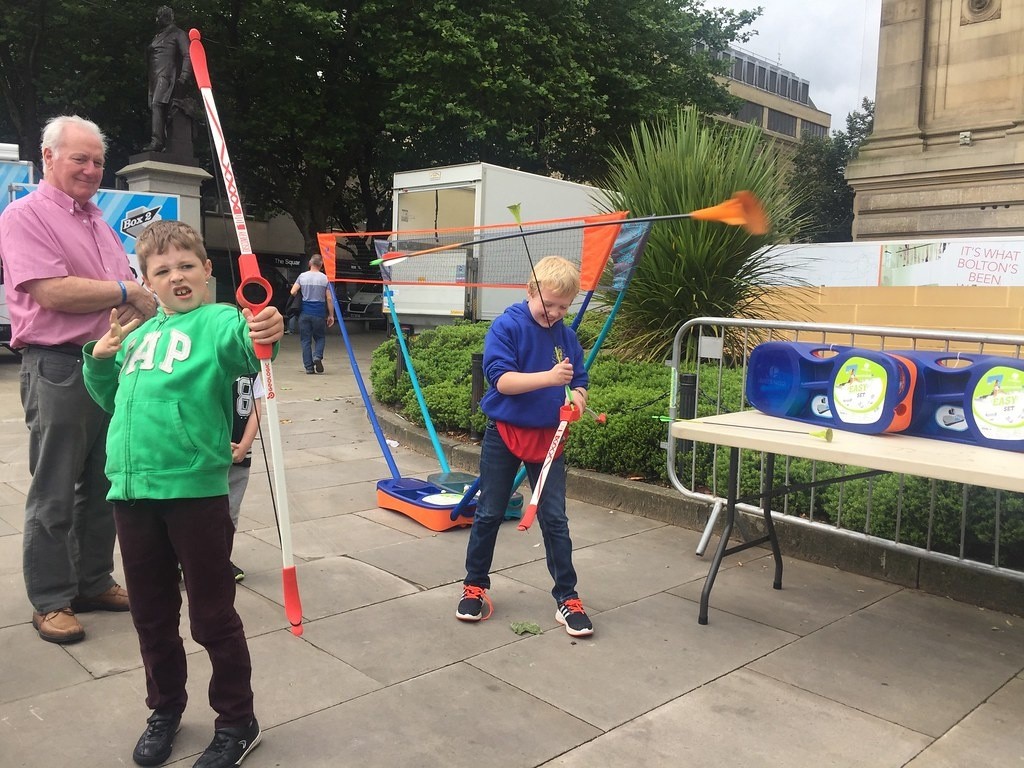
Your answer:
118,281,127,304
571,388,584,398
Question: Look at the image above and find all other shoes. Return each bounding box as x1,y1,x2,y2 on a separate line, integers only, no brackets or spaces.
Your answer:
306,370,315,374
313,356,324,372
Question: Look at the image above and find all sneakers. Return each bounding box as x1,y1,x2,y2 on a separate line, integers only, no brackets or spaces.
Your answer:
456,584,494,622
133,711,182,766
555,598,593,636
231,562,244,581
192,718,261,768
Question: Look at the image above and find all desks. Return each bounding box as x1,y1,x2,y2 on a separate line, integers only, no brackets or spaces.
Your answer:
671,410,1024,625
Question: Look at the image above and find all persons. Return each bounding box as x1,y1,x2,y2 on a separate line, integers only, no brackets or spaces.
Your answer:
227,370,265,583
290,253,335,375
82,220,285,768
0,116,159,644
455,255,594,637
284,313,297,334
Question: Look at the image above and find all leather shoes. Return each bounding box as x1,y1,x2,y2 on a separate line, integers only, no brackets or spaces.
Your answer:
33,608,85,643
28,342,83,358
75,584,130,613
